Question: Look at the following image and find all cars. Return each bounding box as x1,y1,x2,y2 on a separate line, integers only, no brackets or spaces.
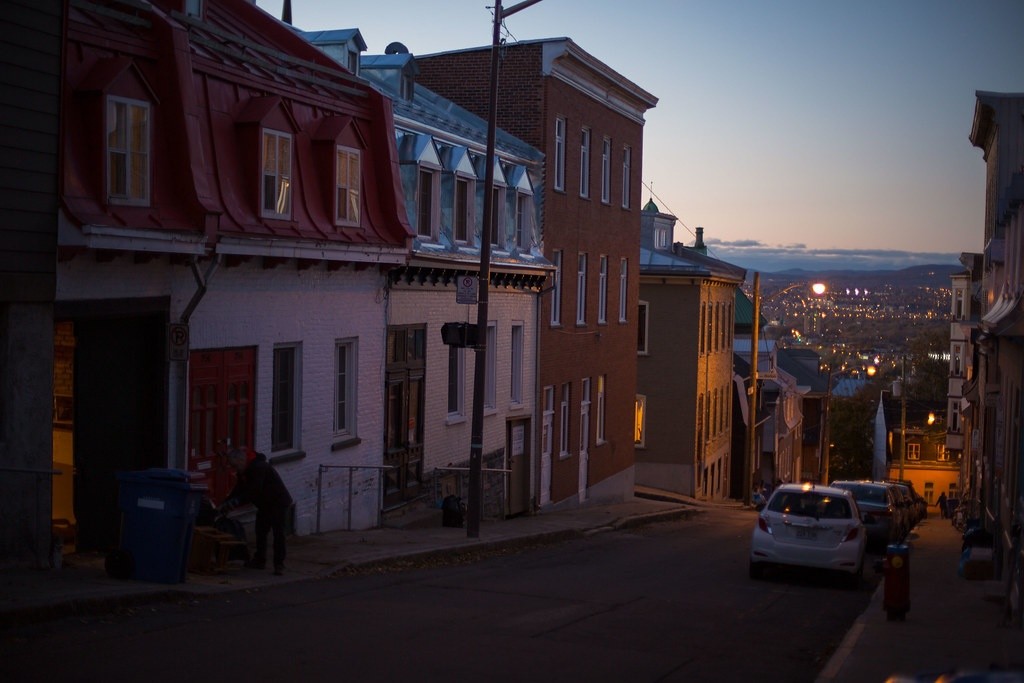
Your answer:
746,483,876,585
830,479,927,549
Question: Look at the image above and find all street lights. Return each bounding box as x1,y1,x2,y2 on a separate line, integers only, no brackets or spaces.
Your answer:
744,271,825,507
820,363,875,486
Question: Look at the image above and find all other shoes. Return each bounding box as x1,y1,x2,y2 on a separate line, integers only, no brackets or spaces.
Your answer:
252,558,265,569
275,561,284,569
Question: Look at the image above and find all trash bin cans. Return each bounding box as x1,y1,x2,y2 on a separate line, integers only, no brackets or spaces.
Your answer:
947,498,959,518
105,467,208,584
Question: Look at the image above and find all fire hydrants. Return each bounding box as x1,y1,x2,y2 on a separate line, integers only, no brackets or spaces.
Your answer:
873,541,912,624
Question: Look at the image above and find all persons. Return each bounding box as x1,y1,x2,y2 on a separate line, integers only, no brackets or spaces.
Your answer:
219,447,294,575
935,492,947,518
